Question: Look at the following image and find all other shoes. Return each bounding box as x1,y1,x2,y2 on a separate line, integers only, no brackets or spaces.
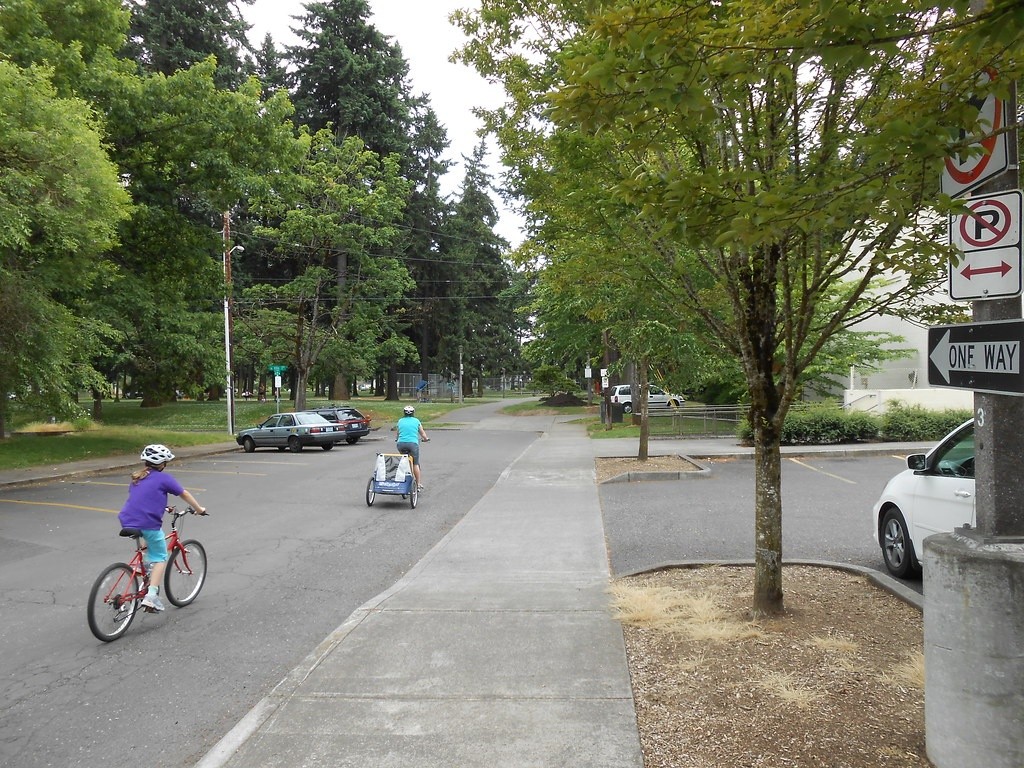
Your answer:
417,485,423,492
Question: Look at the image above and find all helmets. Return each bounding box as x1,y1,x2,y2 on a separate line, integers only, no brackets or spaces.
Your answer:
139,444,176,464
404,406,415,414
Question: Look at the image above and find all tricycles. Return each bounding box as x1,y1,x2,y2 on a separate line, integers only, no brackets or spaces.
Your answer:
366,438,430,509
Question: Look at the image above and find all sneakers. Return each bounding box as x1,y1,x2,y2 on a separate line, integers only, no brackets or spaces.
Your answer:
139,581,165,611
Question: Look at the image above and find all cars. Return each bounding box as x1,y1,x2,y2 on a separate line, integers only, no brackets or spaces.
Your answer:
236,412,347,453
870,418,976,577
127,387,143,398
610,383,685,415
300,404,370,444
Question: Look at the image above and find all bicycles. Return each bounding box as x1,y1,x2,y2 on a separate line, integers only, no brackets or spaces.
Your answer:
87,505,210,641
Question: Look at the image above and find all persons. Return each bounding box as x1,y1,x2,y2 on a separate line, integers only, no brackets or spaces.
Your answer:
395,406,428,493
118,445,205,611
235,383,278,402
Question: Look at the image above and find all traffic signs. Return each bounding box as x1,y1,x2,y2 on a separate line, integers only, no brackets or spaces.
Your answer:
925,320,1024,396
